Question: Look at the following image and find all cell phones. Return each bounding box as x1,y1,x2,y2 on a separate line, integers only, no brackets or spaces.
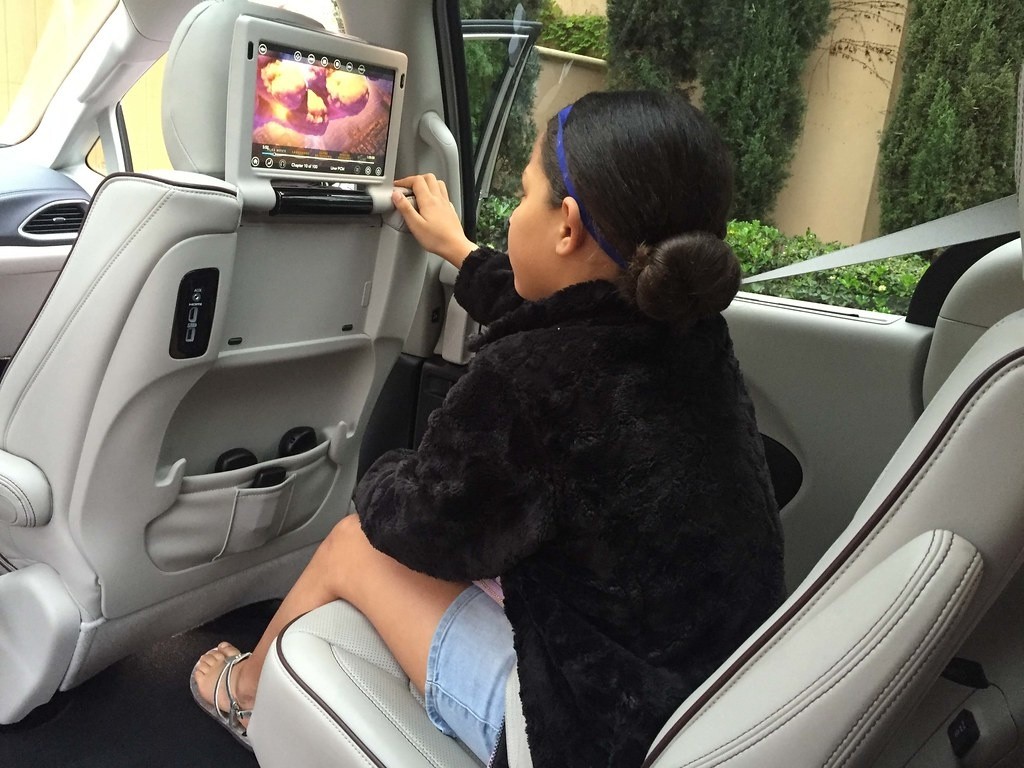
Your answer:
253,468,286,487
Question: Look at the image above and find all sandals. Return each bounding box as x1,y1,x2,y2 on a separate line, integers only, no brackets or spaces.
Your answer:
190,646,254,751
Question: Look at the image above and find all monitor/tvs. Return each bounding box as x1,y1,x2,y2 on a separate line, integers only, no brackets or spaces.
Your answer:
223,16,409,214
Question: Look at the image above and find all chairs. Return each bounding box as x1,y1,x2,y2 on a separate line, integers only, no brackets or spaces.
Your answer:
245,238,1024,768
0,0,427,725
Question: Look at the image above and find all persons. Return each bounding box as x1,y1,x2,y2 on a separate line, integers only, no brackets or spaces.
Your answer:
193,92,787,768
